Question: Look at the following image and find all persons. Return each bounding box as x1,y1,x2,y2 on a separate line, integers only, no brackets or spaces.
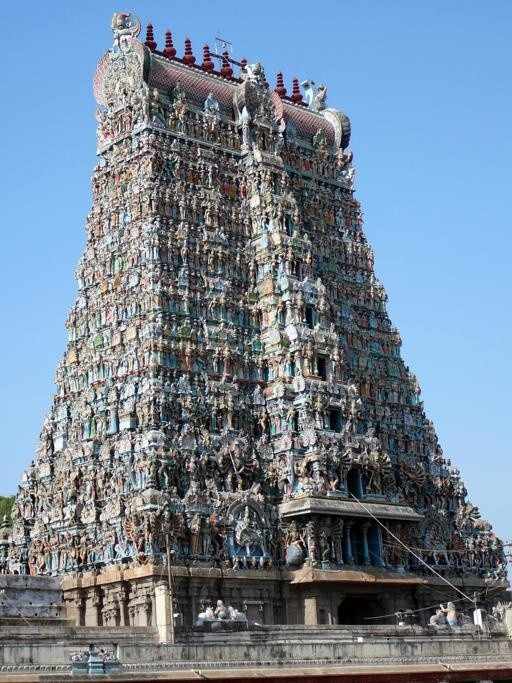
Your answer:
0,60,512,635
